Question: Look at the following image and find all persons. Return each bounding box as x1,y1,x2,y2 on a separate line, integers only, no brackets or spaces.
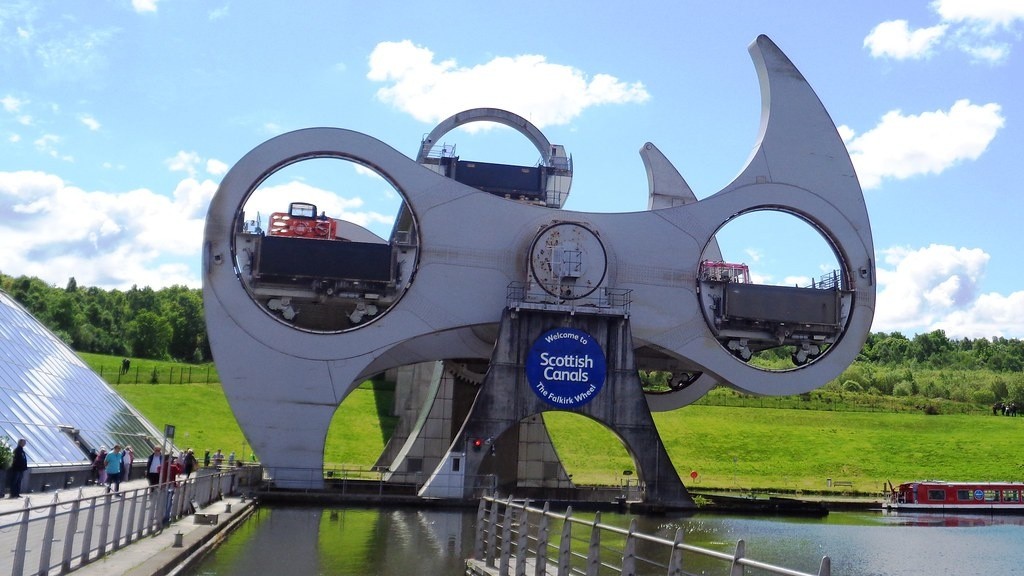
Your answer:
76,441,134,498
214,450,225,471
204,449,210,468
1001,403,1017,417
9,439,28,497
147,446,196,500
993,404,997,415
228,452,235,468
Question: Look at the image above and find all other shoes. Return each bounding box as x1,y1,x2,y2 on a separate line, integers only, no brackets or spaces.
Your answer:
115,494,121,497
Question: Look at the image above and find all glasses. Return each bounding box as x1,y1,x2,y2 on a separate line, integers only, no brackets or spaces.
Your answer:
154,449,161,452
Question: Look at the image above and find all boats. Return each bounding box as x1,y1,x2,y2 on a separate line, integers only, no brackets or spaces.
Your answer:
765,497,830,517
879,480,1024,513
